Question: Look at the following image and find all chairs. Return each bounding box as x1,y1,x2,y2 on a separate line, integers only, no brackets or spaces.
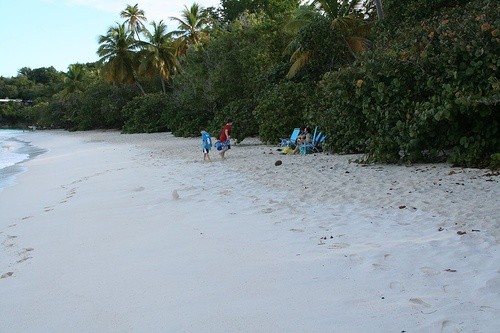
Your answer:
301,125,327,152
281,127,301,149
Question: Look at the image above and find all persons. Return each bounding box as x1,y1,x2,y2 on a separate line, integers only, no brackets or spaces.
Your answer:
291,127,313,155
288,125,306,151
218,118,234,158
200,128,212,161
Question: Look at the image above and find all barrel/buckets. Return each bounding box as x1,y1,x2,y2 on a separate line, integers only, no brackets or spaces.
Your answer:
214,141,222,150
